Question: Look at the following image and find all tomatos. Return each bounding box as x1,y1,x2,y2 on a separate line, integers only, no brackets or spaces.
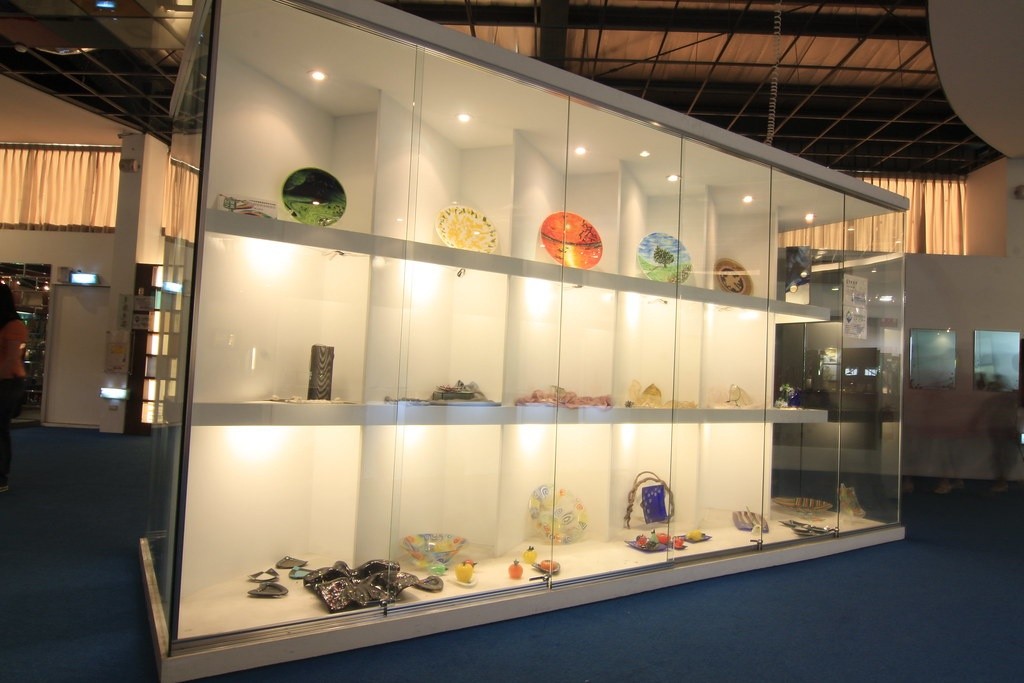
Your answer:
657,533,683,547
508,546,557,579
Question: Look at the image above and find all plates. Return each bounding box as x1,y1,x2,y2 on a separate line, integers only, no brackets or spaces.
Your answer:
280,165,346,225
435,206,498,254
623,539,667,553
771,495,833,516
541,211,603,270
637,232,693,285
733,510,770,532
529,484,589,545
678,535,712,542
713,258,753,297
642,484,668,525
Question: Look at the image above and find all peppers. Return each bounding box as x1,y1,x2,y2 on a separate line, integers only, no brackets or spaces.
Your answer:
456,561,473,582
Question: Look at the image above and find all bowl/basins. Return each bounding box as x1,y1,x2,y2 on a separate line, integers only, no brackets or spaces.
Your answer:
398,532,468,565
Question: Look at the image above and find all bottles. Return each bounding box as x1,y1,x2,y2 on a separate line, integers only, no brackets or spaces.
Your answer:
649,529,658,543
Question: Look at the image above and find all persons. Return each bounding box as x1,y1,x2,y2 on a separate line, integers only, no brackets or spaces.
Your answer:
0,283,29,494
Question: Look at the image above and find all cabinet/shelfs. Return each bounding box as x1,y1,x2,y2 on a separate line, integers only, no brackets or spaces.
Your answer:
141,0,910,683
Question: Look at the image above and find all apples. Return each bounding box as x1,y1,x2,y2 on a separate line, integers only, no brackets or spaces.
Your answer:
636,534,646,545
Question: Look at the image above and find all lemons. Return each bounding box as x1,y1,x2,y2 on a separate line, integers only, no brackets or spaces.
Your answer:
685,530,702,541
